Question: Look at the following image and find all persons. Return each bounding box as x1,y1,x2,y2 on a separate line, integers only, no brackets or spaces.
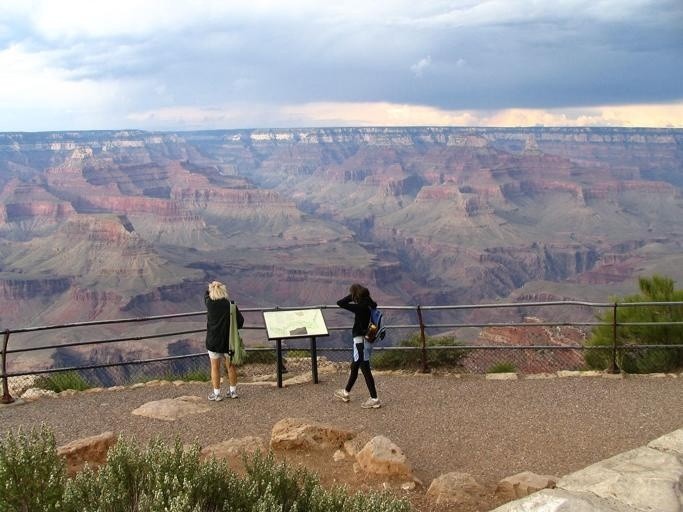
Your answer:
334,283,386,408
204,281,244,401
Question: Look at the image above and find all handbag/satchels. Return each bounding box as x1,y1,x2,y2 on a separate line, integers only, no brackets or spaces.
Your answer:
223,298,250,368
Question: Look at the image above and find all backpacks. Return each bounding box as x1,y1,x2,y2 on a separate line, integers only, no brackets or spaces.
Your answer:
363,299,388,345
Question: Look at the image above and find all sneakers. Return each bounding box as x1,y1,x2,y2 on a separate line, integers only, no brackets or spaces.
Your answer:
332,387,351,403
359,396,381,409
206,391,224,402
224,390,238,399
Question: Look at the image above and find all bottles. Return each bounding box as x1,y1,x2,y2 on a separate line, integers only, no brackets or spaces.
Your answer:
364,325,376,340
